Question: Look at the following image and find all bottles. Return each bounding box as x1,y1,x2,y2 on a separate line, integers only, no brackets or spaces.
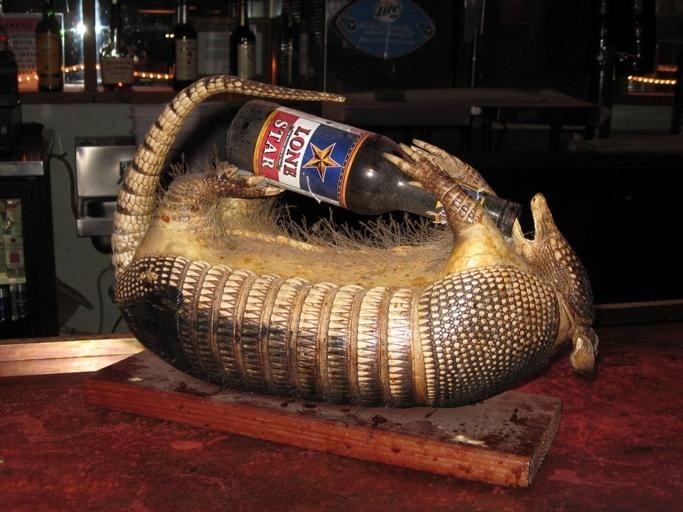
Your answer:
2,199,25,278
230,1,257,80
37,2,62,91
173,2,198,90
226,99,523,237
99,0,133,92
